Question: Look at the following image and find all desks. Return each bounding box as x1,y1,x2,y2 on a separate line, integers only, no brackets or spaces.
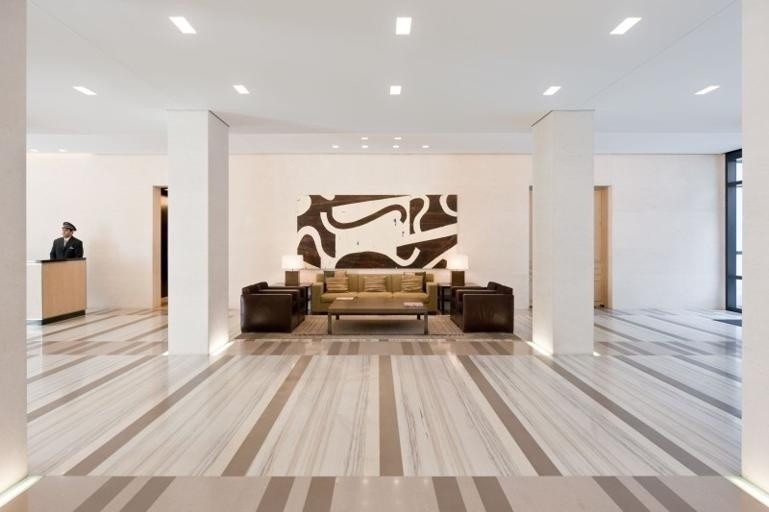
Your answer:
437,280,480,315
271,280,314,314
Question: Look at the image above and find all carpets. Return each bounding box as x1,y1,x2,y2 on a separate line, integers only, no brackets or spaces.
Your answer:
233,313,522,341
714,320,742,327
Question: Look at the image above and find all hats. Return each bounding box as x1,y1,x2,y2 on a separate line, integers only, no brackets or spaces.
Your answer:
61,221,76,231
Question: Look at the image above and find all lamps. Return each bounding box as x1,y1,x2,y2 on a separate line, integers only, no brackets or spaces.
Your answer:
280,253,303,287
446,253,469,287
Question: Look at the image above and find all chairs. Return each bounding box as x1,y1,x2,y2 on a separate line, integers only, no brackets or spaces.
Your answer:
238,281,307,333
452,282,514,333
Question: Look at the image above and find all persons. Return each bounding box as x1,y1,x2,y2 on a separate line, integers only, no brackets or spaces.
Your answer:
48,220,83,259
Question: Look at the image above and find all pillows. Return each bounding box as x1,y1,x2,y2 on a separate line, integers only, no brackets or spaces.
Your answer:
403,272,425,293
364,276,387,293
401,275,423,292
323,270,346,279
324,277,348,292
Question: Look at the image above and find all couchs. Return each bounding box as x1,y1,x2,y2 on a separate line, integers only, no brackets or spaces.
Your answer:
310,269,436,313
